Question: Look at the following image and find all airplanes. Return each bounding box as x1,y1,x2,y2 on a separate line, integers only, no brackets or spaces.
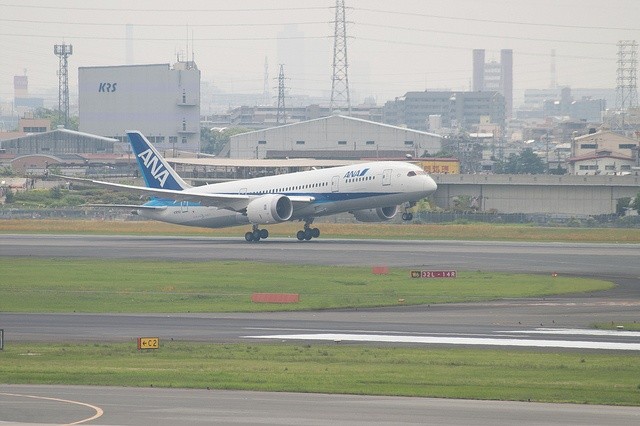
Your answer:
52,131,438,241
